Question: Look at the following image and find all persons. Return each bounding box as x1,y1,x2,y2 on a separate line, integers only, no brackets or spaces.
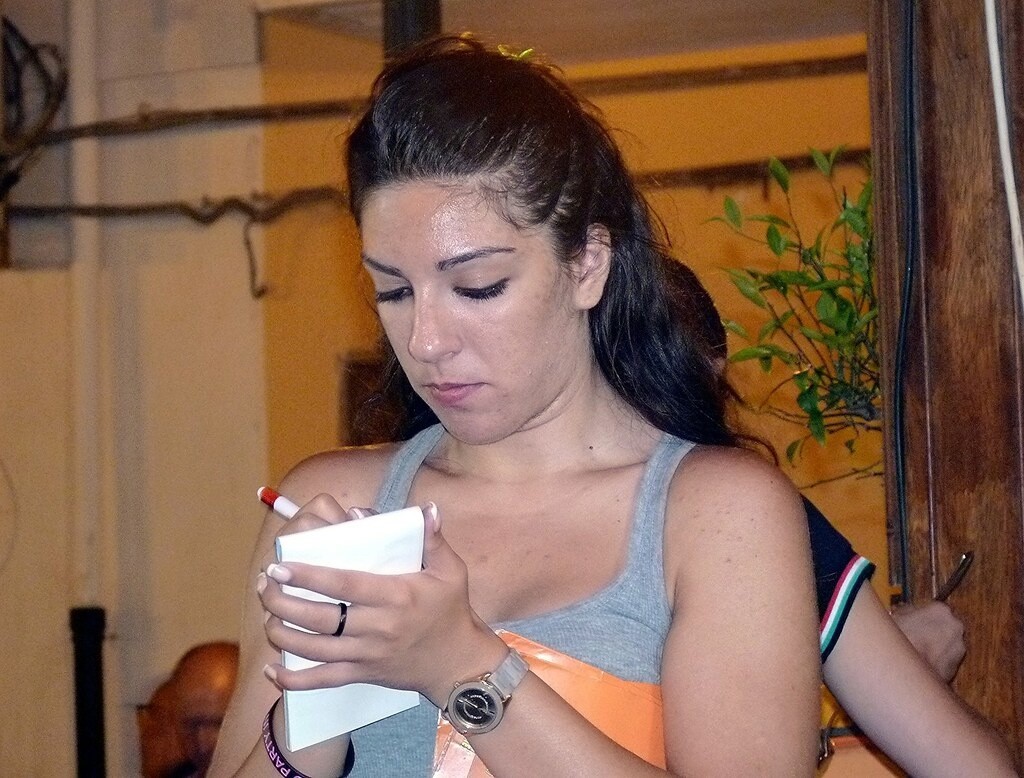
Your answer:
206,34,1023,778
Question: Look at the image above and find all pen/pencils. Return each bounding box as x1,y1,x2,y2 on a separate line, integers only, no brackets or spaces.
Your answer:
256,484,300,520
936,550,977,601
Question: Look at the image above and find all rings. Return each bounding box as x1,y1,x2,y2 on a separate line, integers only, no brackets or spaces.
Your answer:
332,603,348,639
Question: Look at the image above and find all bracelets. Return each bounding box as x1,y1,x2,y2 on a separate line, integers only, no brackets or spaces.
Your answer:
263,699,355,778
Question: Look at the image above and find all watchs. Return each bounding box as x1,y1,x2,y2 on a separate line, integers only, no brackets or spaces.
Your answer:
440,646,531,737
